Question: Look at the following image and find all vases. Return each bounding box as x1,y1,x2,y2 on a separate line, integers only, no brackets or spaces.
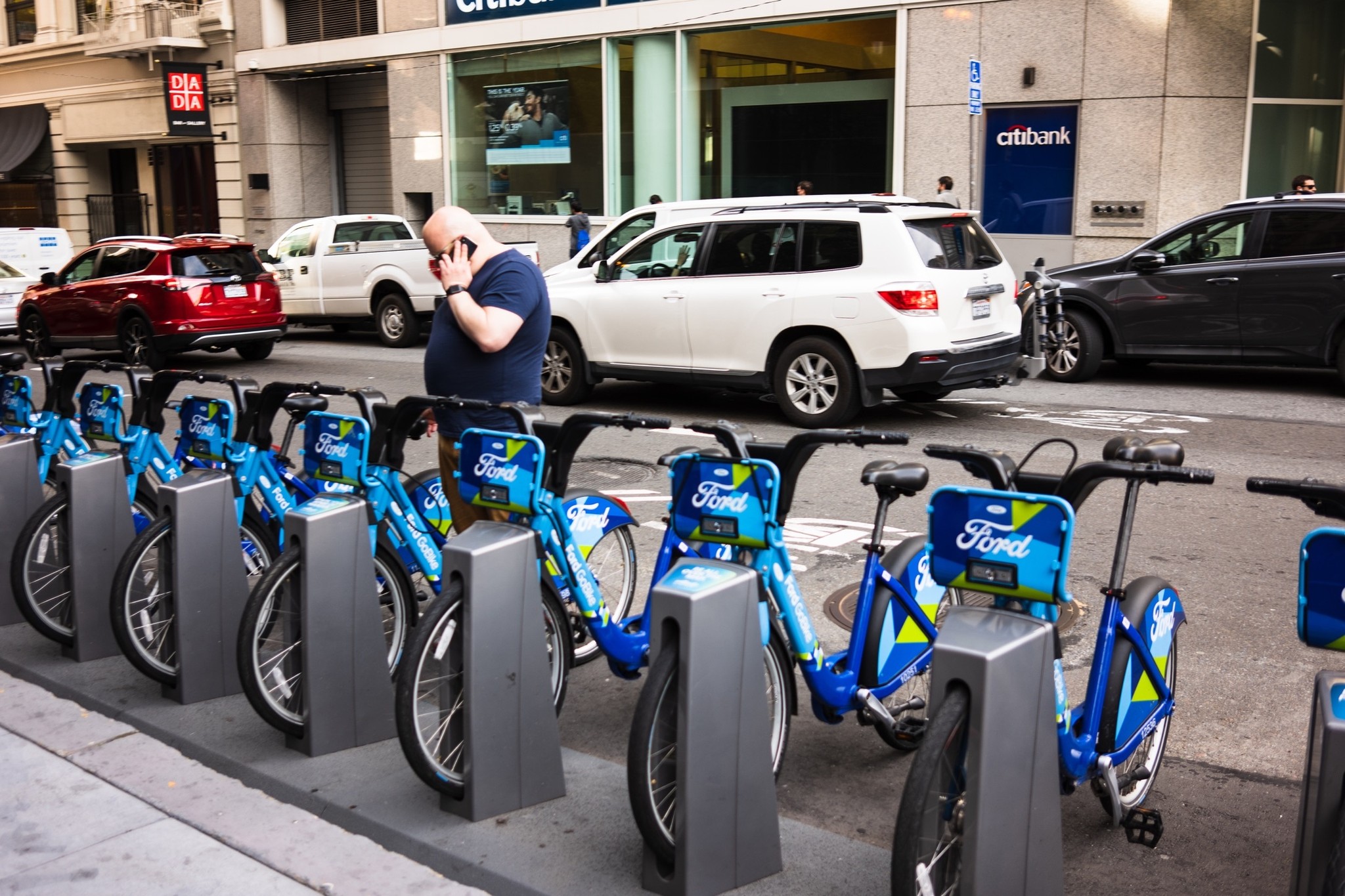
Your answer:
497,206,507,214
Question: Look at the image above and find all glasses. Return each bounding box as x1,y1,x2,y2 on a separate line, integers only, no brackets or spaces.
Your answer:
1302,185,1316,189
429,235,466,260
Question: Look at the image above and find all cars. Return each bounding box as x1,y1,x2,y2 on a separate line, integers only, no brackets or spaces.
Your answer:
1015,192,1345,390
0,259,43,341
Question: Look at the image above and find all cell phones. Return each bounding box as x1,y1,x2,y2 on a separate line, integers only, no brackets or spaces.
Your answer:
448,236,477,262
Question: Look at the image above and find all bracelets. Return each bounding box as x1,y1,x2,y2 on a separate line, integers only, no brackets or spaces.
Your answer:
673,264,682,270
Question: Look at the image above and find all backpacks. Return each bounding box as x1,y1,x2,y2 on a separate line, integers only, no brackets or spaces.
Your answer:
576,229,590,250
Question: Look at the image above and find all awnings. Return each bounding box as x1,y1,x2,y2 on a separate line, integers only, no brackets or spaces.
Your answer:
0,103,50,182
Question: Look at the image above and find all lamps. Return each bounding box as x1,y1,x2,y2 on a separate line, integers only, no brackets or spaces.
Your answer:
1024,67,1035,85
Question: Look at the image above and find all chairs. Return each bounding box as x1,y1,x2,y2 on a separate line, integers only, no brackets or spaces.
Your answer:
97,256,129,278
771,241,796,273
690,240,746,276
1244,222,1271,259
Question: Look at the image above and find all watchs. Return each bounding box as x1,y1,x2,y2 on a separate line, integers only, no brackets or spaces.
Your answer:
446,285,468,297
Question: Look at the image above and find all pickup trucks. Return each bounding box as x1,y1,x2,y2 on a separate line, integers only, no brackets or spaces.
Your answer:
256,212,542,349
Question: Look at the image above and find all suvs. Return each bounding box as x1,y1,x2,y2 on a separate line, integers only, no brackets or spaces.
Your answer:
541,190,1026,429
15,232,287,372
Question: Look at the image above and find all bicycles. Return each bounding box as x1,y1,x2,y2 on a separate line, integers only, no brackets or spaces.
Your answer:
1,350,650,738
625,418,958,874
394,394,738,800
1245,475,1345,660
888,434,1217,896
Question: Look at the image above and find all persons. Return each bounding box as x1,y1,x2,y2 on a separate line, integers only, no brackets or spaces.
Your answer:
797,181,814,195
1291,175,1317,195
935,176,961,209
672,245,691,277
649,194,664,204
996,181,1023,234
565,202,590,259
494,87,569,149
419,205,550,535
